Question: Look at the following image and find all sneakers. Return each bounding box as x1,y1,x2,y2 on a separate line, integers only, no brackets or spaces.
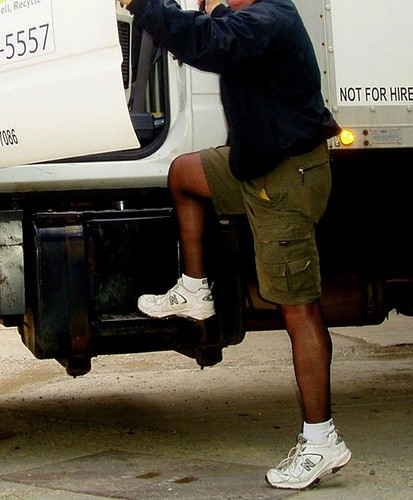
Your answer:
265,430,351,490
137,278,216,321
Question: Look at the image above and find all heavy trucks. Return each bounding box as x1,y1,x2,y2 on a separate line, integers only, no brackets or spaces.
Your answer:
0,0,413,379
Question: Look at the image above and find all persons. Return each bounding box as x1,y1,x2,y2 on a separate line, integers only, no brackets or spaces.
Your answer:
118,0,353,491
